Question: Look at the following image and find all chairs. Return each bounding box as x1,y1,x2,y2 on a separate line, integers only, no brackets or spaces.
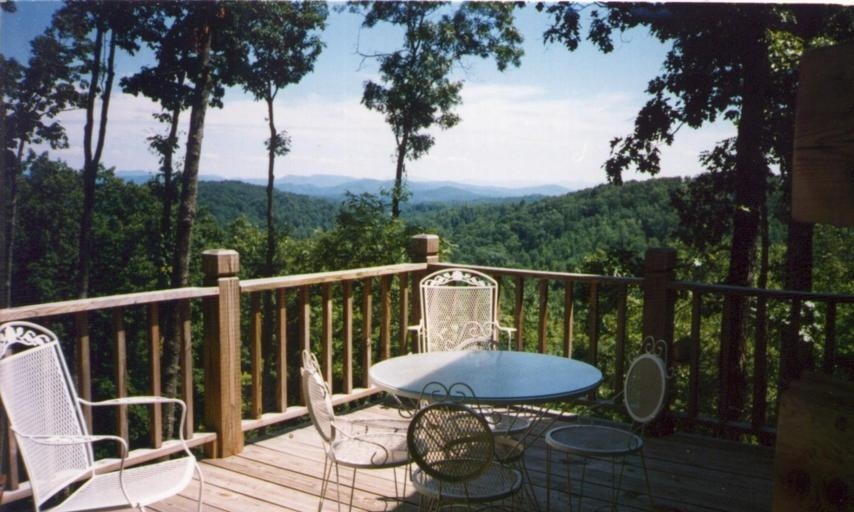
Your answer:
541,333,670,509
0,317,201,512
365,348,606,509
403,268,521,431
404,379,525,509
294,346,429,509
